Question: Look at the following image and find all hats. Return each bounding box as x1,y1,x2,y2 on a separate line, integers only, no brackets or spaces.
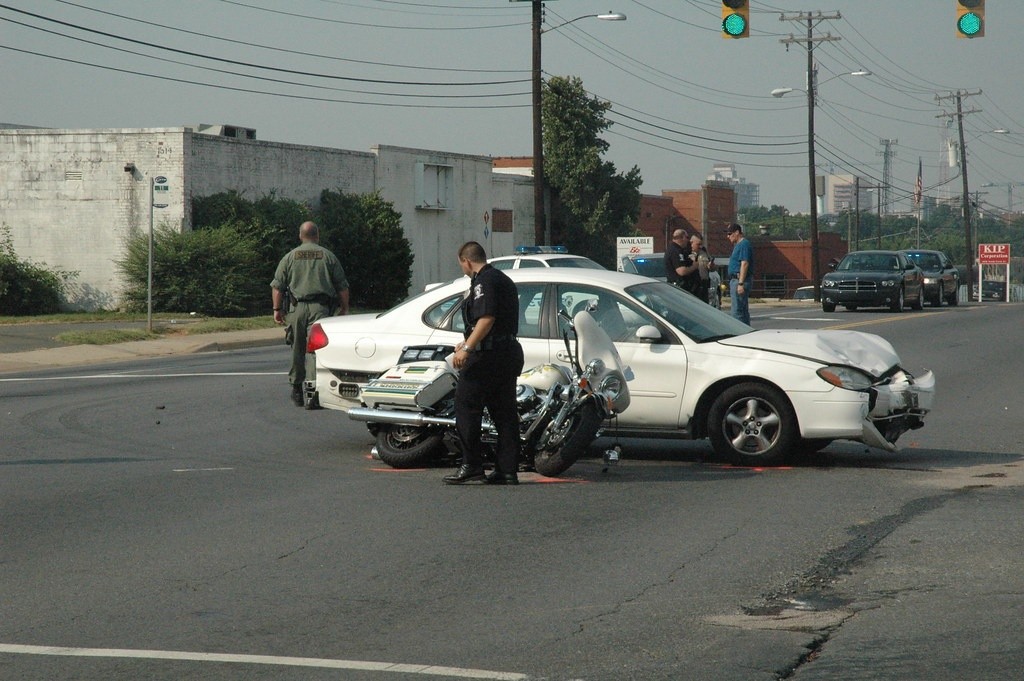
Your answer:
722,223,741,232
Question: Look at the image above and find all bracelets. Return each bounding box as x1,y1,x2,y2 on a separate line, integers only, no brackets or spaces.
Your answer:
738,283,744,285
273,307,282,311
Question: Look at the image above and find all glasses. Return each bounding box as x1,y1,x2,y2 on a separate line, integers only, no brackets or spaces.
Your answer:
727,233,732,235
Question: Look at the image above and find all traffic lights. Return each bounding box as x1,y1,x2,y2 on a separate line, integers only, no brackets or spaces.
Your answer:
954,0,985,38
720,0,750,38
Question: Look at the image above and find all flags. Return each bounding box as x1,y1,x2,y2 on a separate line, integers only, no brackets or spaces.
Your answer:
914,161,922,206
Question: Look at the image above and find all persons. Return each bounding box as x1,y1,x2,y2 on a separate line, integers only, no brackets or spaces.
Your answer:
271,222,349,410
726,223,754,327
443,241,523,485
664,228,717,307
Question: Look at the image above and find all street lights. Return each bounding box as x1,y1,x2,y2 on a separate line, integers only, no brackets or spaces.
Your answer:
769,69,874,305
531,10,627,244
960,128,1010,302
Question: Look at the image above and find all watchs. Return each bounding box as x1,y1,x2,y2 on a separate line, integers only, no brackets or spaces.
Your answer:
462,345,473,354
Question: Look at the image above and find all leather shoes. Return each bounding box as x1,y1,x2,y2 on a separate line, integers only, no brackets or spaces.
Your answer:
442,464,486,484
485,470,520,484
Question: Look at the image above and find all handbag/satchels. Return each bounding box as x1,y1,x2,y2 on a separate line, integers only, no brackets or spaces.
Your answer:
676,276,706,299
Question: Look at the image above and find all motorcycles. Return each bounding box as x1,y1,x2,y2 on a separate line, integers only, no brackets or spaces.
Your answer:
349,307,632,477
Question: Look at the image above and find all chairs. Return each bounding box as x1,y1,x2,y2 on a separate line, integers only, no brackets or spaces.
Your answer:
575,299,628,343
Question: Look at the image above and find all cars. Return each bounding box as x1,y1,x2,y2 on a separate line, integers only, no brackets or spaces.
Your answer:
896,248,960,307
972,280,1007,302
793,286,830,303
822,251,928,313
423,252,653,331
302,268,935,466
622,253,724,312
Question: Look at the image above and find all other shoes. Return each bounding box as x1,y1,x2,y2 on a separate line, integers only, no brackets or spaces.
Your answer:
290,384,304,407
304,391,324,410
